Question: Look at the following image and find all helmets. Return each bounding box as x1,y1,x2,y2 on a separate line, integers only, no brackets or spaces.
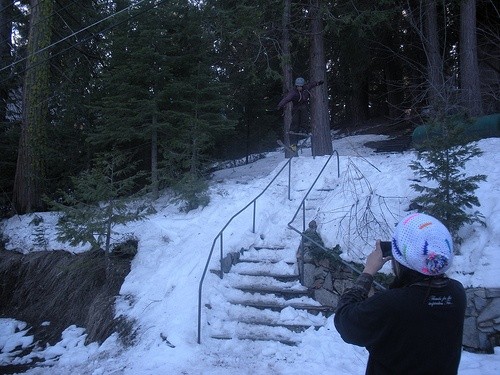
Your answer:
295,78,305,86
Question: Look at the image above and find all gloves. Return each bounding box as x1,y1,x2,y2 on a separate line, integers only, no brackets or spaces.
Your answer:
316,80,324,85
276,106,282,110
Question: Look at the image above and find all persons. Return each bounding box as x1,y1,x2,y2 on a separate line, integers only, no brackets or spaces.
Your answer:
334,213,467,375
277,77,324,135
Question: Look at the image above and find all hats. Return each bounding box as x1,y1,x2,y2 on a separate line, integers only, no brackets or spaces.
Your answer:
393,214,453,275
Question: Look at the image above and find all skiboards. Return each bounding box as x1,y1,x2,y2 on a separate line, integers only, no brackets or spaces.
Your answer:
288,131,313,137
277,139,299,157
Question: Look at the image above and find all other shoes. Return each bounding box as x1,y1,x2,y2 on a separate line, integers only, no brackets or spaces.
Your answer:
301,128,304,133
293,129,299,133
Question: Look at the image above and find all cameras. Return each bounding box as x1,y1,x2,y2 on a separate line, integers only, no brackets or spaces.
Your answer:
381,241,392,258
